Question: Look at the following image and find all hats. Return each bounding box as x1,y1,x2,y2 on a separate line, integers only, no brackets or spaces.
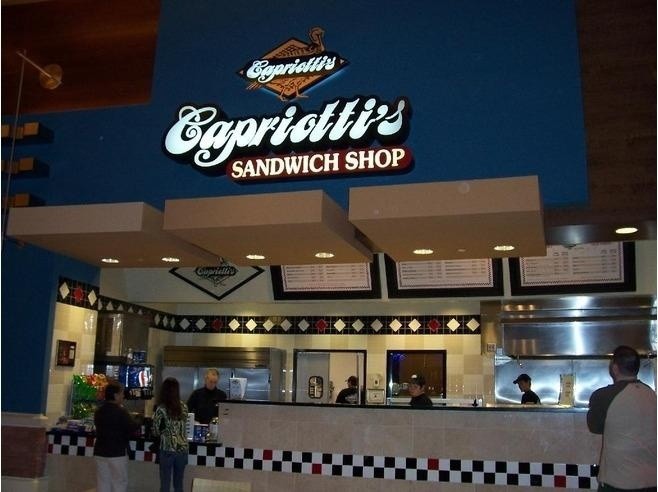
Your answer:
513,374,530,384
345,376,356,385
408,374,425,385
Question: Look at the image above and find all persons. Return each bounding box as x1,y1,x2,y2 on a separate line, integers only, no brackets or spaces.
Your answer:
401,374,433,407
92,380,141,492
150,378,189,492
186,368,229,427
336,375,363,405
587,345,656,492
513,374,541,404
